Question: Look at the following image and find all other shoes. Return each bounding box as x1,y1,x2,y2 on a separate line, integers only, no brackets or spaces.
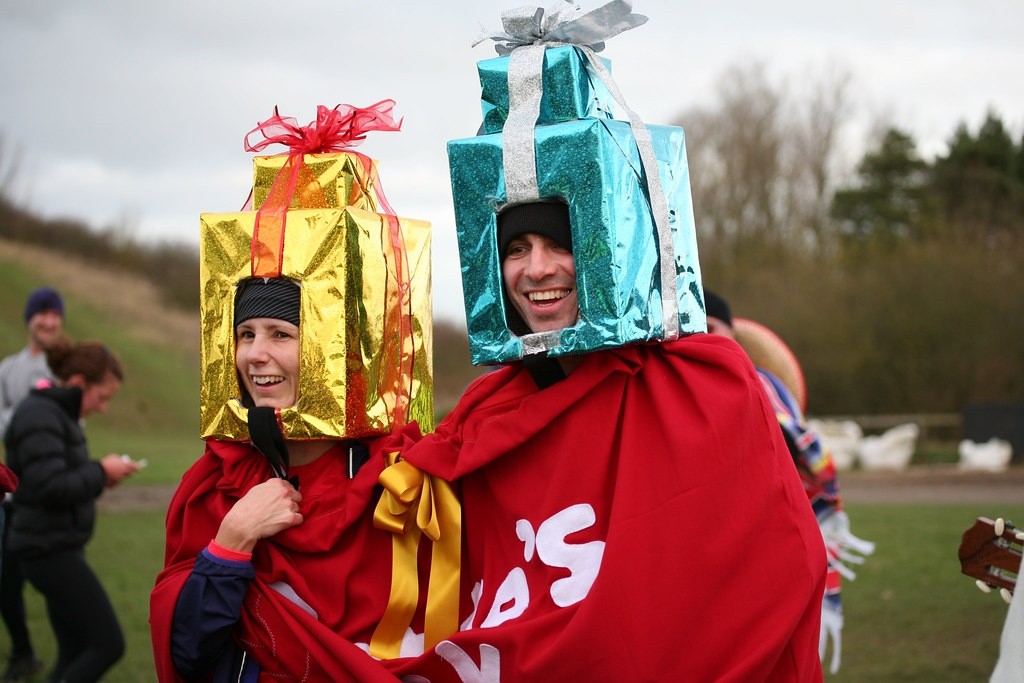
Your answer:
3,655,41,682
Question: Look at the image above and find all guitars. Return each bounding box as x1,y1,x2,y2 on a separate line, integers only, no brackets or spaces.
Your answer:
957,515,1024,603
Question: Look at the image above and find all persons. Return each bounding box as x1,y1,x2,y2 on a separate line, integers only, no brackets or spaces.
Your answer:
404,201,828,683
149,275,432,683
5,342,140,683
0,288,88,683
703,289,875,677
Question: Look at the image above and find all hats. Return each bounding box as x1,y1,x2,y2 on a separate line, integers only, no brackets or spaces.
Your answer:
234,277,300,326
24,288,62,326
703,288,731,326
497,198,573,269
